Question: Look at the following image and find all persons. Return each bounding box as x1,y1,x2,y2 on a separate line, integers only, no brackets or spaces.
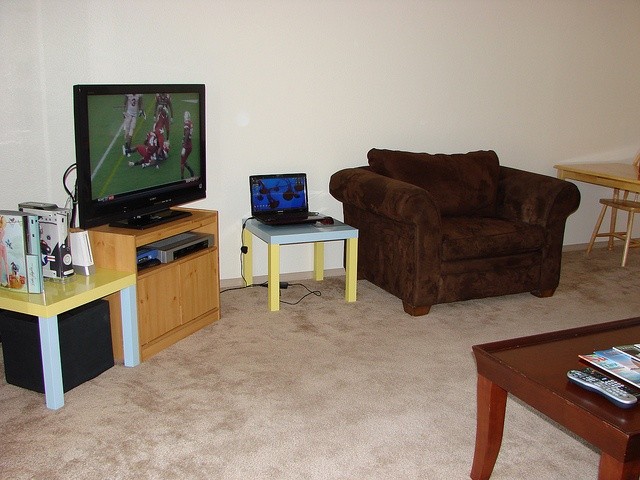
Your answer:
153,94,173,140
122,142,169,167
153,104,169,159
143,132,156,166
180,111,195,179
122,94,147,157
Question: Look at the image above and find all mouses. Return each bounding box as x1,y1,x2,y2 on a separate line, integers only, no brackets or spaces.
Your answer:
321,216,334,225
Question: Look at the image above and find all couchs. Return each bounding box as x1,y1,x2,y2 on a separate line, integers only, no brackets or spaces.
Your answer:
329,150,580,316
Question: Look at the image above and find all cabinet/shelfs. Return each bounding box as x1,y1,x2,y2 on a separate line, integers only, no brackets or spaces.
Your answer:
66,206,220,367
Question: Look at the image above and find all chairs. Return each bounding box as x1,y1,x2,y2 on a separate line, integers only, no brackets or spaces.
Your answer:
586,153,640,268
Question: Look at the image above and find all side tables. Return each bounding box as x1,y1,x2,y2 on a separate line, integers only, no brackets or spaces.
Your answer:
241,213,359,312
0,265,140,411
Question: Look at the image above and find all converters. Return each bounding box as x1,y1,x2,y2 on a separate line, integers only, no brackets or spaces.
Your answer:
280,282,288,288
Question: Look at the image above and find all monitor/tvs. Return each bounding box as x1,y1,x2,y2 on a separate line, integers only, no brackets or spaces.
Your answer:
74,83,207,230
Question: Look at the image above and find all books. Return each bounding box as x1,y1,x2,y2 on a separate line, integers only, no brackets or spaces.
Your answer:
577,343,640,388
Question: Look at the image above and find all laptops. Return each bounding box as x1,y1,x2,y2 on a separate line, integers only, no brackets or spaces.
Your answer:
249,173,317,227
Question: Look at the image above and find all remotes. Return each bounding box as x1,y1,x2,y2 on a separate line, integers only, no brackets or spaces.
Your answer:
565,369,638,412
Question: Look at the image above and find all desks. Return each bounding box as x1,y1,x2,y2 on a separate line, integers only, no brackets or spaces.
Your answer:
554,161,639,250
